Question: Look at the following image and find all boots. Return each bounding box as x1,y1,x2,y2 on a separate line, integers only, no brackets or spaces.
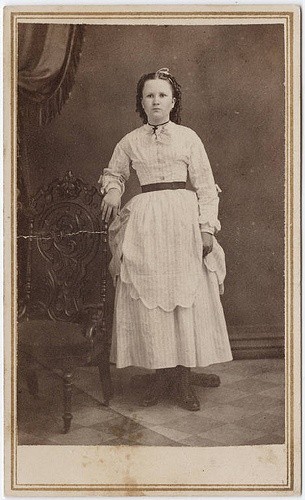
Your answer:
170,363,200,411
141,368,172,406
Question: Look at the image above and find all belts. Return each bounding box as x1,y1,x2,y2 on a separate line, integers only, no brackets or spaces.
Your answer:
141,182,186,192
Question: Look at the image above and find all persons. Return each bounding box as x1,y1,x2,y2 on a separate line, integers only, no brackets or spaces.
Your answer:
99,67,234,413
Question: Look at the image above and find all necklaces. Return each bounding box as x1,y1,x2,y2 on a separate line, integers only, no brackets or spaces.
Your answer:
147,120,170,134
